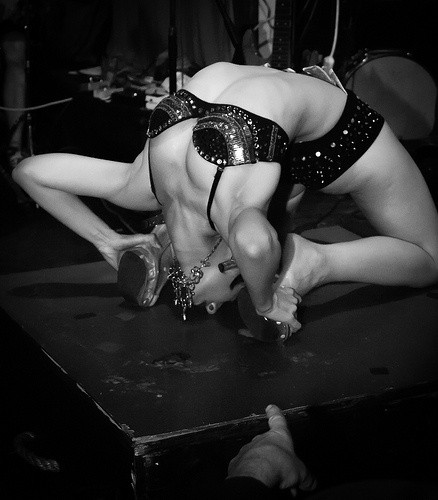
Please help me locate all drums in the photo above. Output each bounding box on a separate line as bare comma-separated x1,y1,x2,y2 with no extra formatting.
342,48,438,150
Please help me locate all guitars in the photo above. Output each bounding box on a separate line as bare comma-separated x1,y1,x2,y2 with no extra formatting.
240,0,299,70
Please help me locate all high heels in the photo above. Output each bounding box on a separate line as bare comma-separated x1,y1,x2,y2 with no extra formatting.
217,256,292,344
119,247,160,309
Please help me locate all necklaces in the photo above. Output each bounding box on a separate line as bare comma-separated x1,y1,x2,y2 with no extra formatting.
168,239,222,318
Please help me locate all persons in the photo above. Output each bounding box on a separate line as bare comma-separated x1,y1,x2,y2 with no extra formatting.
38,43,176,236
12,61,438,349
216,403,321,500
